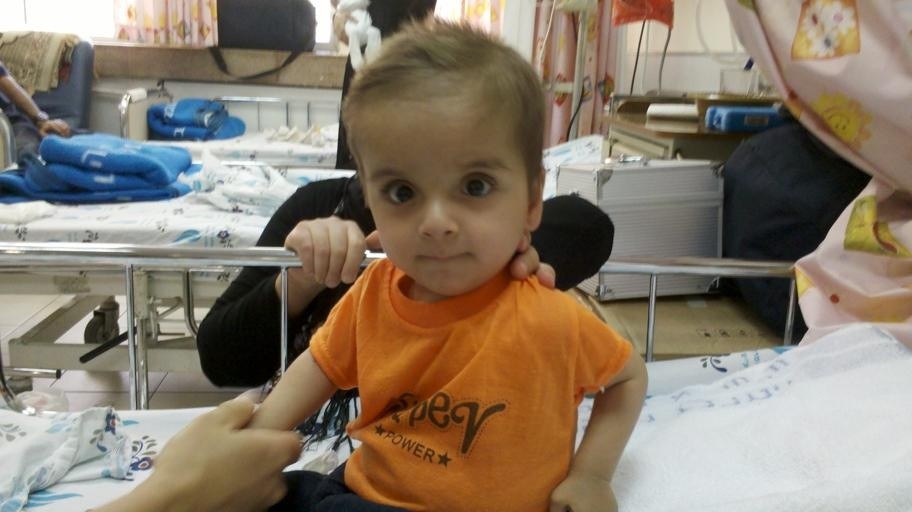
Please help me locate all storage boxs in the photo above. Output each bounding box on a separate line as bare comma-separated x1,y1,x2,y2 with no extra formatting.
556,160,726,304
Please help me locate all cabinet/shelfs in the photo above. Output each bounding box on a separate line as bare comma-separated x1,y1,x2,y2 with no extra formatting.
605,89,781,161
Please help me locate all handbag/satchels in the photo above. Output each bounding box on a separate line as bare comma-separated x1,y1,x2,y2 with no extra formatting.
216,0,316,51
722,123,872,331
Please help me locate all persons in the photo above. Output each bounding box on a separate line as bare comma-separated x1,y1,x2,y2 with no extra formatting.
330,0,381,74
197,170,615,398
0,58,69,140
84,396,301,512
244,12,648,512
333,0,437,169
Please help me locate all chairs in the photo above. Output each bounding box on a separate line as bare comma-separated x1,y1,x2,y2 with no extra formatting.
0,29,94,161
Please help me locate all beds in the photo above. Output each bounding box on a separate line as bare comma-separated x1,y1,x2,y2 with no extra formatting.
119,87,340,165
0,111,637,410
0,242,912,512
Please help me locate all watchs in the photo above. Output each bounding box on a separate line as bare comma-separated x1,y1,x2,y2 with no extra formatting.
33,112,49,125
